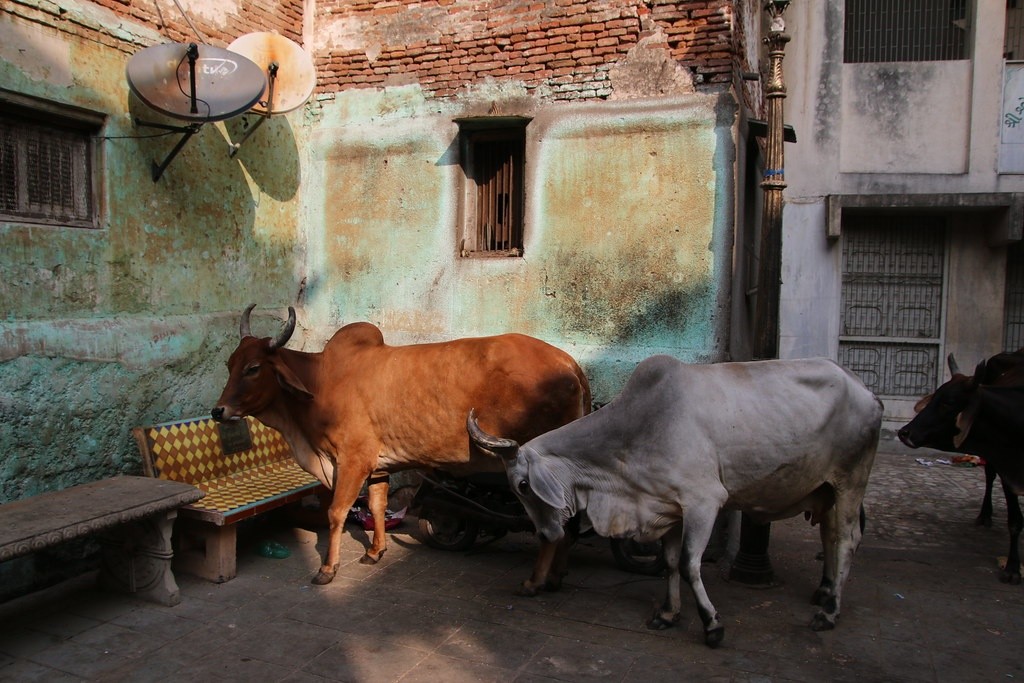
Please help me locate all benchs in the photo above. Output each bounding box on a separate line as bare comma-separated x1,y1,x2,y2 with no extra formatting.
0,474,207,607
132,412,327,582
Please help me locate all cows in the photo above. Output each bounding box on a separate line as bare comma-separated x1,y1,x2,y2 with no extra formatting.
899,346,1024,587
212,303,888,648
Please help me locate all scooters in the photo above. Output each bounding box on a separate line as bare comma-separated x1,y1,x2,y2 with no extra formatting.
416,392,666,574
